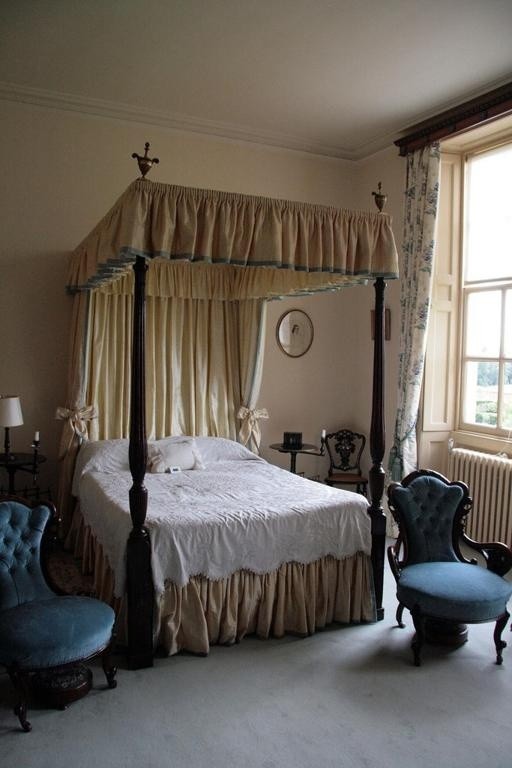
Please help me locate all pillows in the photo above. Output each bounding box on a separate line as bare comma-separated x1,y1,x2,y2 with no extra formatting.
163,436,262,463
148,439,204,472
80,438,129,474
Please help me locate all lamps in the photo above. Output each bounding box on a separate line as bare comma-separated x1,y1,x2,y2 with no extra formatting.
0,395,25,462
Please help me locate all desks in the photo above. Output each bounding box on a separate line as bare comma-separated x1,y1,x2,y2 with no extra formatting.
0,452,47,493
269,443,317,474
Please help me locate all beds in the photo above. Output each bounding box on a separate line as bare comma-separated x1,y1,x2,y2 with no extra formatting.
66,140,399,672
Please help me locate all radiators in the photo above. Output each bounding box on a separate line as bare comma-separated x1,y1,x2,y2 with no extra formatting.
446,436,512,549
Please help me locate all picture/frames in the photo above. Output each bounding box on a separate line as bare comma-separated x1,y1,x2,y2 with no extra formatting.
276,309,314,357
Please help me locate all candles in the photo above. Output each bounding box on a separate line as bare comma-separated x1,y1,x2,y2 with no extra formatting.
34,430,40,442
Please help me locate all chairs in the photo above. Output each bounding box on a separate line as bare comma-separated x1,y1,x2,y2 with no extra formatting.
0,493,117,732
324,430,368,496
387,468,512,668
